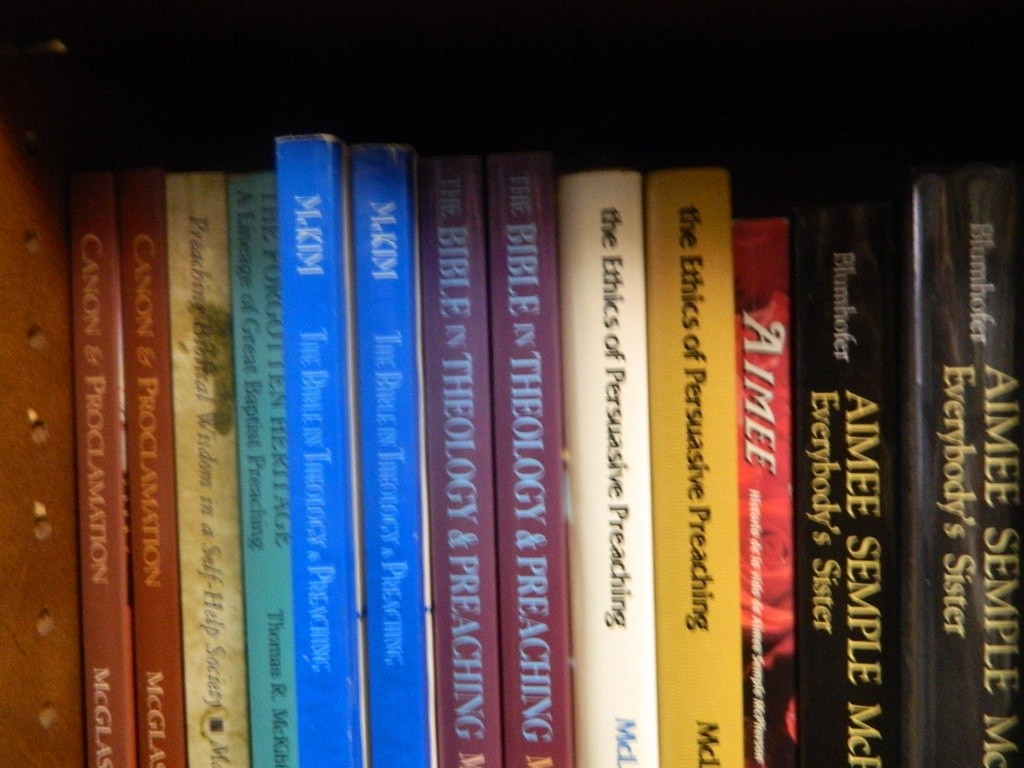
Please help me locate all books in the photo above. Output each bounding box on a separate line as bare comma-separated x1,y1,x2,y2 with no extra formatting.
0,105,1024,768
